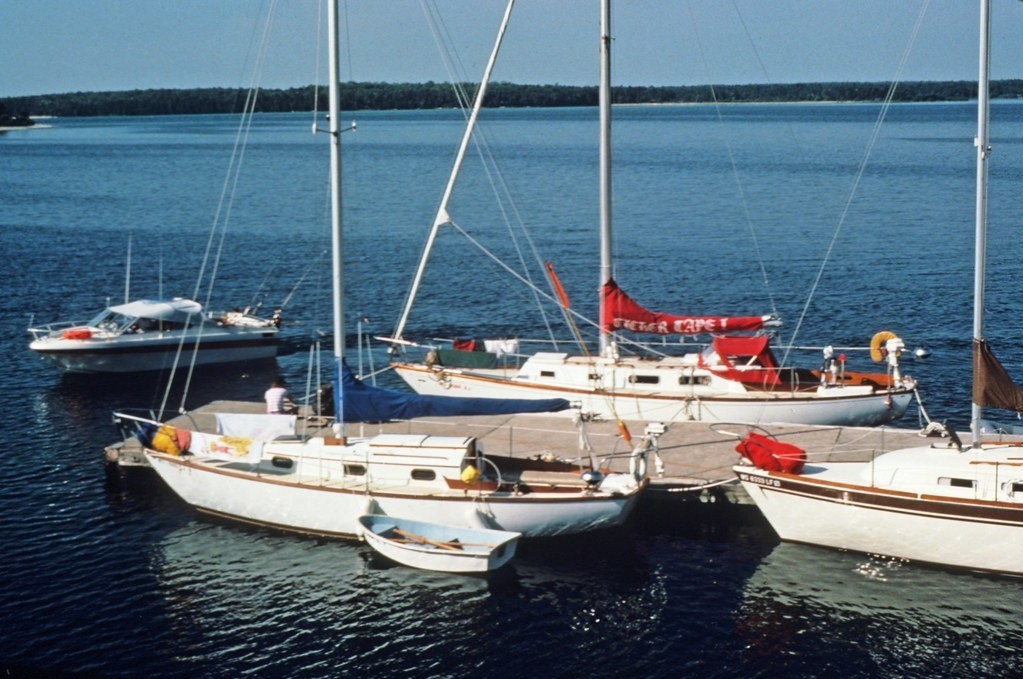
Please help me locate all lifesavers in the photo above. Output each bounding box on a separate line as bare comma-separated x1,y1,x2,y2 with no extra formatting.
870,331,901,362
63,330,92,339
630,447,648,489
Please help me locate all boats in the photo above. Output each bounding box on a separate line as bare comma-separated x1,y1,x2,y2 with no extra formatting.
356,514,522,575
26,230,330,376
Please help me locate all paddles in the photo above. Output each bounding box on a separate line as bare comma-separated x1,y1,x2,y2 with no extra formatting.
388,529,494,551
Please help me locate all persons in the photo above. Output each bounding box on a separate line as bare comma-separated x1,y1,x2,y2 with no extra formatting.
263,378,298,415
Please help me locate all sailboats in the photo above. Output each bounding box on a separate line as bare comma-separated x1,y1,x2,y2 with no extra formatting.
390,2,925,424
103,1,654,543
729,2,1022,585
